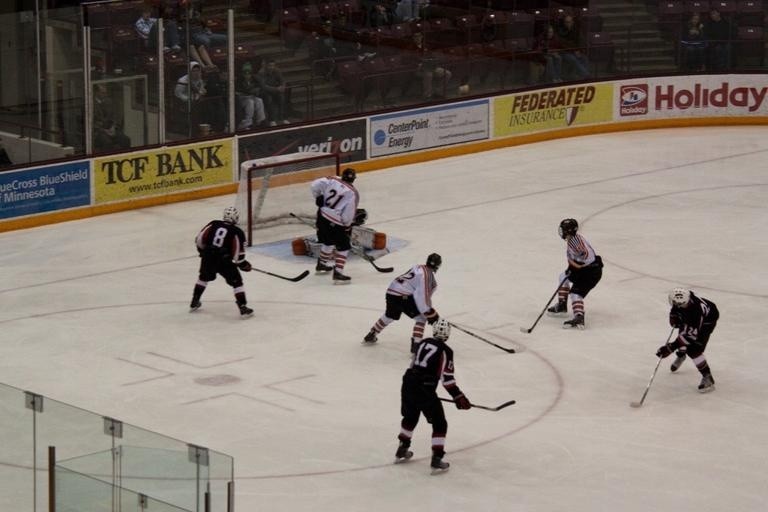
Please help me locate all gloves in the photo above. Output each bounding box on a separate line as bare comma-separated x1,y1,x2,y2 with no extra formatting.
424,309,439,324
317,195,324,206
455,393,471,409
670,316,680,327
239,260,251,272
656,343,675,358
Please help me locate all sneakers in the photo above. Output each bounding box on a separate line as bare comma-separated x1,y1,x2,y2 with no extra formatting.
671,352,686,371
316,258,351,280
698,375,714,388
396,440,413,458
548,303,567,312
240,306,253,314
191,301,201,307
431,449,450,469
564,315,584,325
411,338,419,354
365,331,378,342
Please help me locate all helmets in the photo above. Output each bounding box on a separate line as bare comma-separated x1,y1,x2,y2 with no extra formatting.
558,219,578,239
671,288,690,305
223,207,240,224
432,318,450,341
426,254,442,269
342,168,356,183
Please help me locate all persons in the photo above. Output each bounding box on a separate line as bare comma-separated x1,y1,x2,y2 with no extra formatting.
190,207,253,316
310,168,367,281
548,218,604,327
655,287,719,390
396,319,470,469
678,8,739,74
364,253,442,353
81,0,291,153
360,0,592,101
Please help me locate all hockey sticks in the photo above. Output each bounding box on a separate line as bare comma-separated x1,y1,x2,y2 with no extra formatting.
447,322,515,353
519,276,568,334
631,327,677,409
251,266,309,282
435,399,516,412
352,238,394,273
